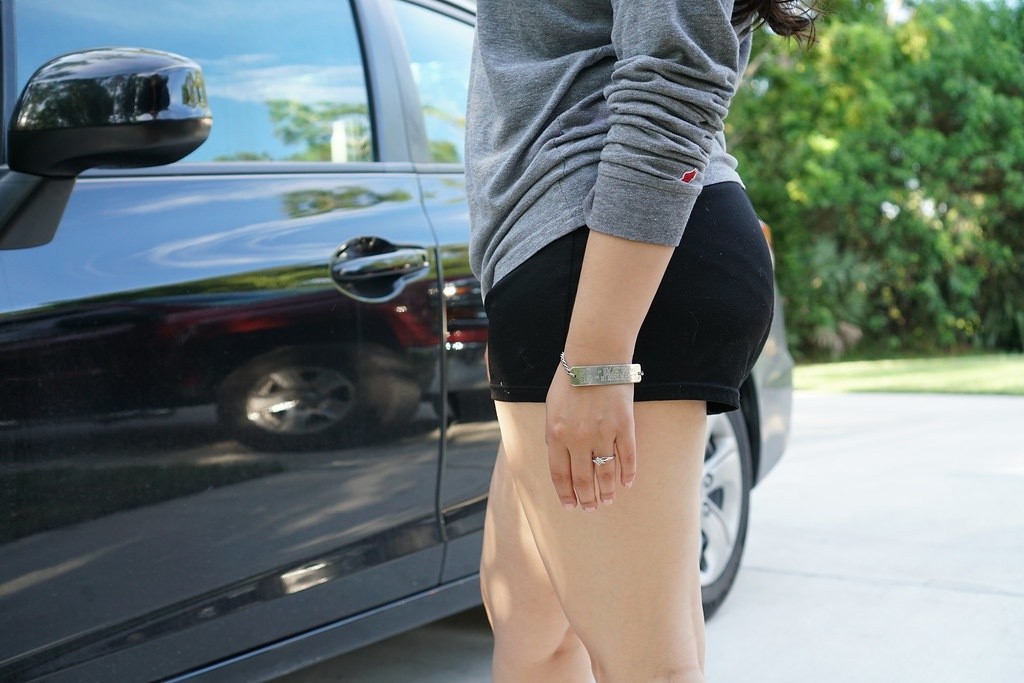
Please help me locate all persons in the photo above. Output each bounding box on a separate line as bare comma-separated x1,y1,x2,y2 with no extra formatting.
464,1,820,683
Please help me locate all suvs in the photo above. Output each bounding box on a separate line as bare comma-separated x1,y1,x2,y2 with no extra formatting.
0,0,795,683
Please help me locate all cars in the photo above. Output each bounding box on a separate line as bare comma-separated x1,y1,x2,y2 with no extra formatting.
43,202,492,452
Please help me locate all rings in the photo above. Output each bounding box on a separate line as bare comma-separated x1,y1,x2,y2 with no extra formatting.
592,453,617,464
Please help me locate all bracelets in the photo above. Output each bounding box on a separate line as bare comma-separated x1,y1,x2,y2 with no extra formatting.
560,352,644,387
